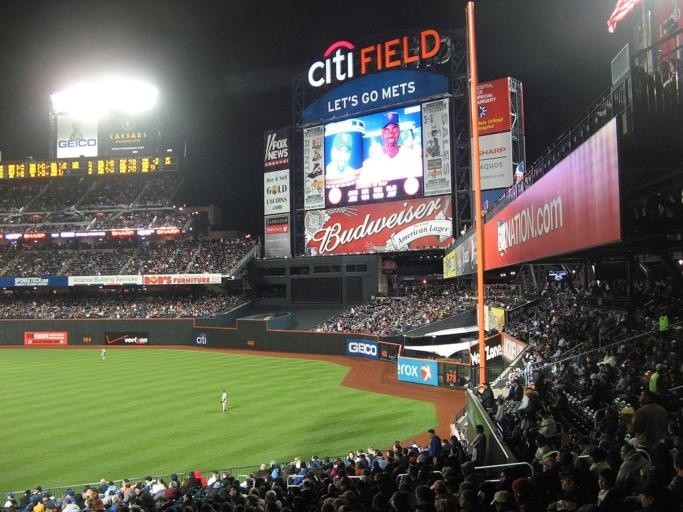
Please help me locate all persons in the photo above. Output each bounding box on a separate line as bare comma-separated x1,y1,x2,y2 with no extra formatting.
220,389,227,412
99,347,106,361
311,278,475,335
0,172,259,319
477,276,683,510
0,427,476,511
324,132,356,180
356,111,423,182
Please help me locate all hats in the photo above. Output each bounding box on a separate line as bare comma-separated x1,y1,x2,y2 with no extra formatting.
489,489,514,506
429,479,445,491
459,461,472,473
557,468,582,481
538,458,554,466
